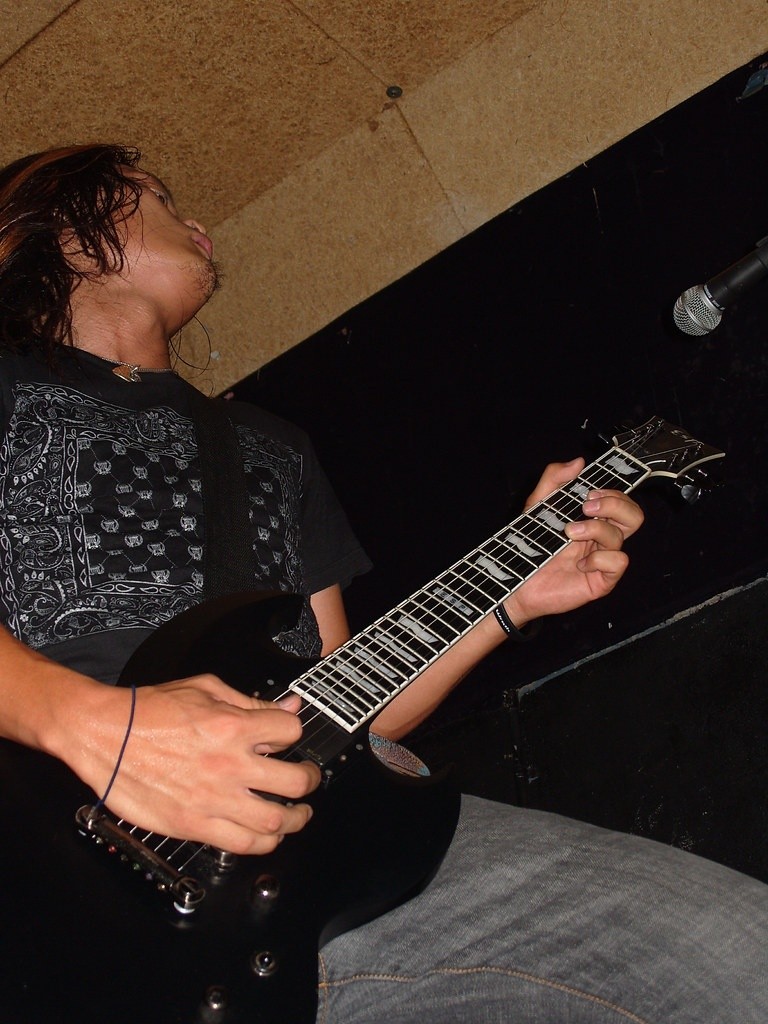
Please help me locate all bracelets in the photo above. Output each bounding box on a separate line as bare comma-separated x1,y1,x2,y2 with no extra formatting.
493,602,543,643
99,685,136,806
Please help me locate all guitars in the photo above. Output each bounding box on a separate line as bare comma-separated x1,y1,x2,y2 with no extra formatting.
0,411,728,1023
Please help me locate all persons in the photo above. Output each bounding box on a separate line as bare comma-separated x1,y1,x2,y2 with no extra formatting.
0,143,644,1024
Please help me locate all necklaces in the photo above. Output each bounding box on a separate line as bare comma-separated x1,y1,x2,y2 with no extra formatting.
65,343,172,383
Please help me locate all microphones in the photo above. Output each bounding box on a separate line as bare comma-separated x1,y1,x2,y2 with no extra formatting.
672,236,768,336
